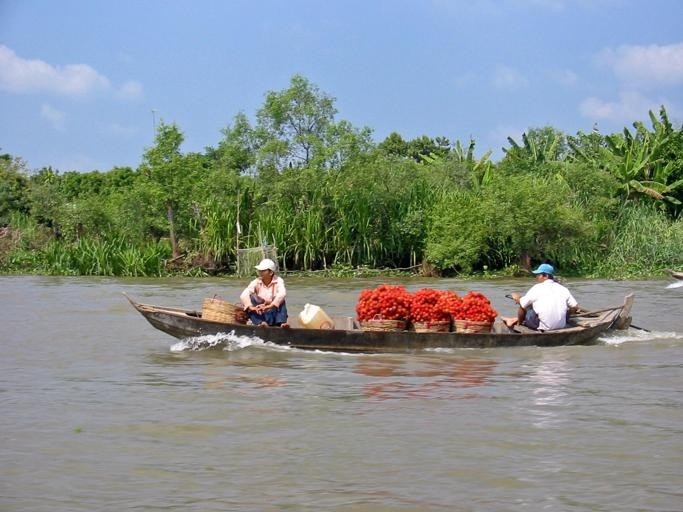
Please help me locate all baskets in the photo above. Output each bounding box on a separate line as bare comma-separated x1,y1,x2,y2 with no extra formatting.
359,318,406,331
411,321,450,333
201,297,249,326
454,320,494,333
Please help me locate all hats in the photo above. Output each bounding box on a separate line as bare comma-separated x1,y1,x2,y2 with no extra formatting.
254,259,276,272
532,264,554,274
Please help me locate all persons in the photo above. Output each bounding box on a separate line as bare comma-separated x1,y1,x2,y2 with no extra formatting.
500,263,581,331
239,258,290,328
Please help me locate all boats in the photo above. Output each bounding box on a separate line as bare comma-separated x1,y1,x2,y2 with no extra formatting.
120,289,635,353
